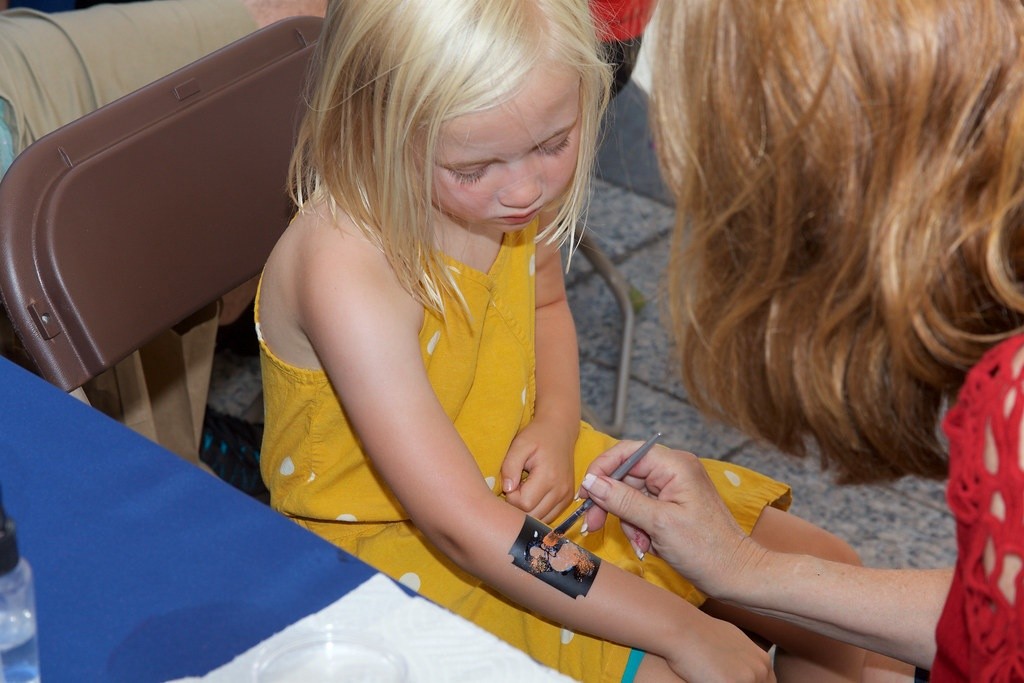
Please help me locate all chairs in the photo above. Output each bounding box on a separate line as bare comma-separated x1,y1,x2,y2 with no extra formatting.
1,15,323,485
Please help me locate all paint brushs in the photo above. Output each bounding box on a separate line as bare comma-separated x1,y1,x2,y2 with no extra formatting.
542,432,664,547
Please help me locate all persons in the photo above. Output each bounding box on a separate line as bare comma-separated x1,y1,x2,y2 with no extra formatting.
253,0,866,682
582,1,654,98
573,0,1023,681
